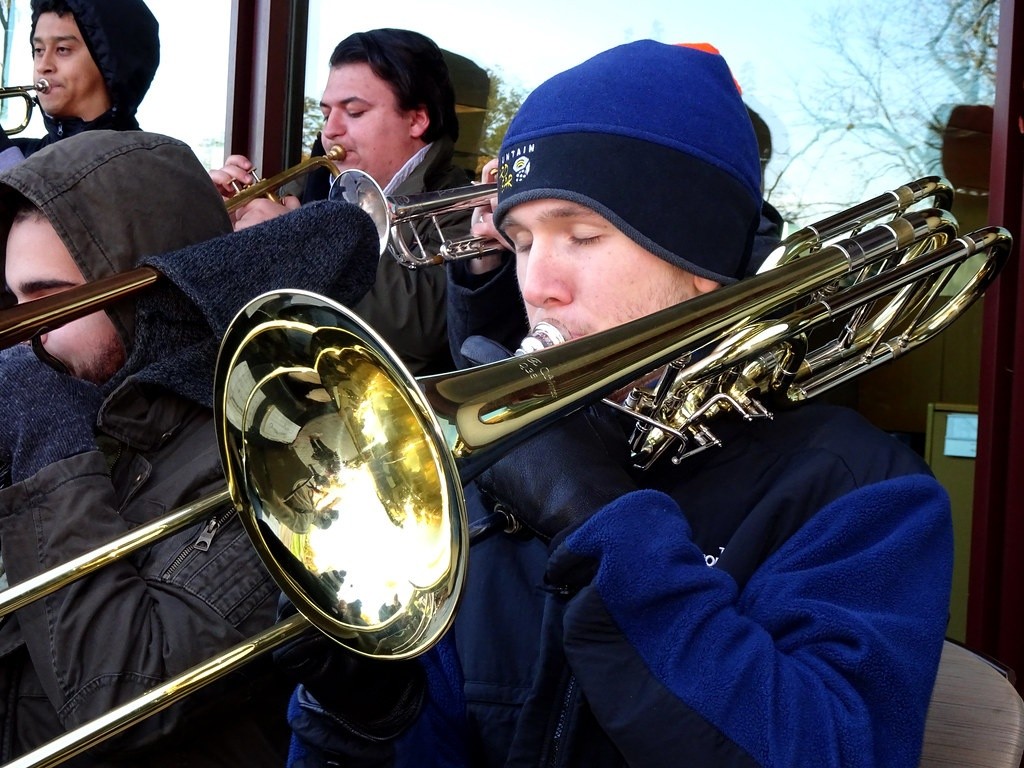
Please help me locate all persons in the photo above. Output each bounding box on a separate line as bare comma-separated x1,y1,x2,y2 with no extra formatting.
445,44,784,371
0,128,282,767
282,39,954,767
0,0,161,175
209,28,479,377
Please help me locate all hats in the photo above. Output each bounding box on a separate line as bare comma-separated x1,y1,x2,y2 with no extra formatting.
492,39,760,284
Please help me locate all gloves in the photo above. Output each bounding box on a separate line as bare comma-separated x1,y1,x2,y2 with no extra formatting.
271,588,429,742
459,334,642,551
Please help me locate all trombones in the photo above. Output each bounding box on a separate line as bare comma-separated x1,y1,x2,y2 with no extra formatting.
0,173,1015,768
0,261,165,358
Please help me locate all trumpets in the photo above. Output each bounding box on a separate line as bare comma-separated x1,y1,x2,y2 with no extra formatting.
323,166,509,274
0,77,54,139
222,141,349,217
